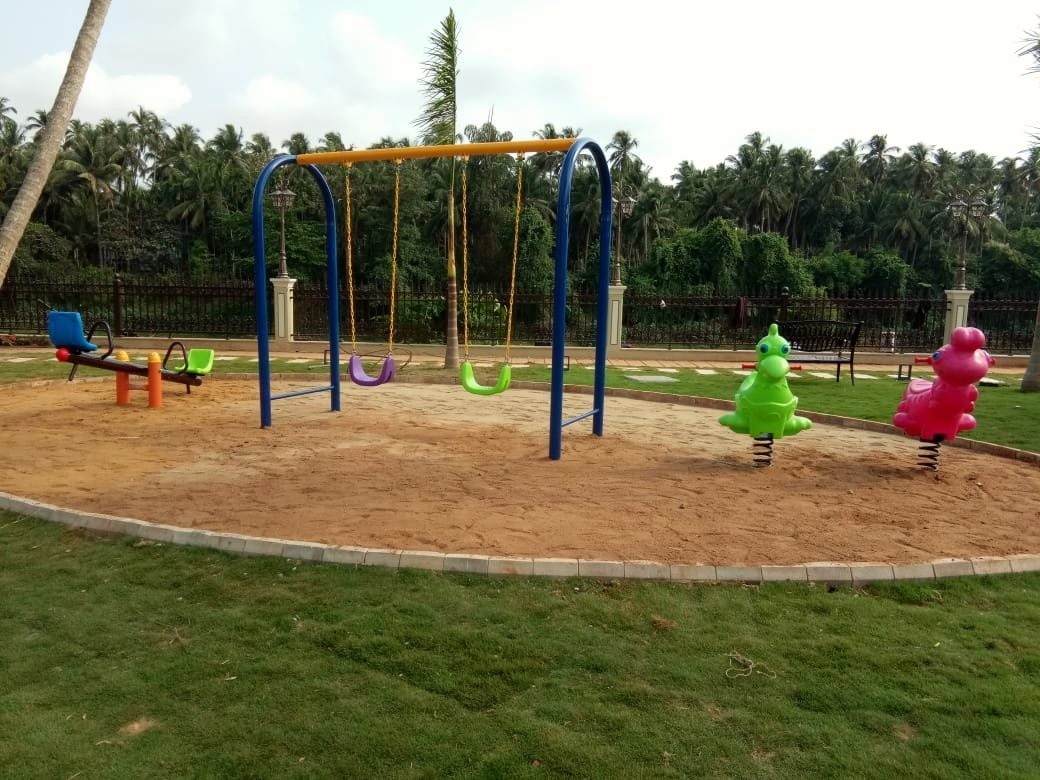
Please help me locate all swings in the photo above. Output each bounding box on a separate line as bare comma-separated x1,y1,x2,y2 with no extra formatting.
456,151,526,396
323,160,404,387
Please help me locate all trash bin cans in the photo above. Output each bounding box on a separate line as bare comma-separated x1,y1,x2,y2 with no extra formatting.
881,331,895,353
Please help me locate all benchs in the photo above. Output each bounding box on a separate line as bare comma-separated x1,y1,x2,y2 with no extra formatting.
776,319,865,386
897,362,931,379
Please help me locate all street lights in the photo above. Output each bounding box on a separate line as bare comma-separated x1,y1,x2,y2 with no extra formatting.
267,178,296,278
610,181,639,286
949,186,990,290
